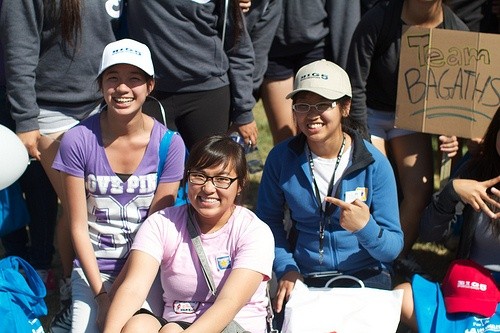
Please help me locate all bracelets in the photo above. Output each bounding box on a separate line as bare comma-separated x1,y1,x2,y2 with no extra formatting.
95,291,107,299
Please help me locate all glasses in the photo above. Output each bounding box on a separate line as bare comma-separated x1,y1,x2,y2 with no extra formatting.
186,169,241,189
292,96,347,113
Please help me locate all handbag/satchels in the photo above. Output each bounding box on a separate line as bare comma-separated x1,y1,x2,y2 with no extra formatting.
0,256,47,333
281,276,405,333
220,320,252,333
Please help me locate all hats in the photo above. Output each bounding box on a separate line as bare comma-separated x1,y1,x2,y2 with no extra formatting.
95,38,155,80
441,259,500,318
285,59,352,100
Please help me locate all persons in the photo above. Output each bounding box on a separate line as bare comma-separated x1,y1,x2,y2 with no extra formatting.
104,136,275,333
51,39,186,333
253,61,402,333
393,108,500,331
0,0,500,313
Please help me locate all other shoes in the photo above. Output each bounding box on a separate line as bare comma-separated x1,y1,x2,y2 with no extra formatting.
394,256,423,280
